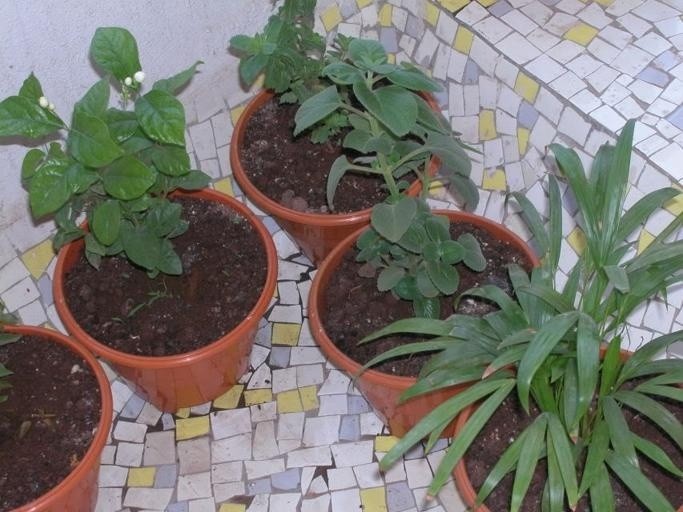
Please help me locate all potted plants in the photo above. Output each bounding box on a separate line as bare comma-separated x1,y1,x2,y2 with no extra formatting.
227,3,448,264
3,26,277,414
292,35,540,445
0,282,115,512
352,121,682,512
